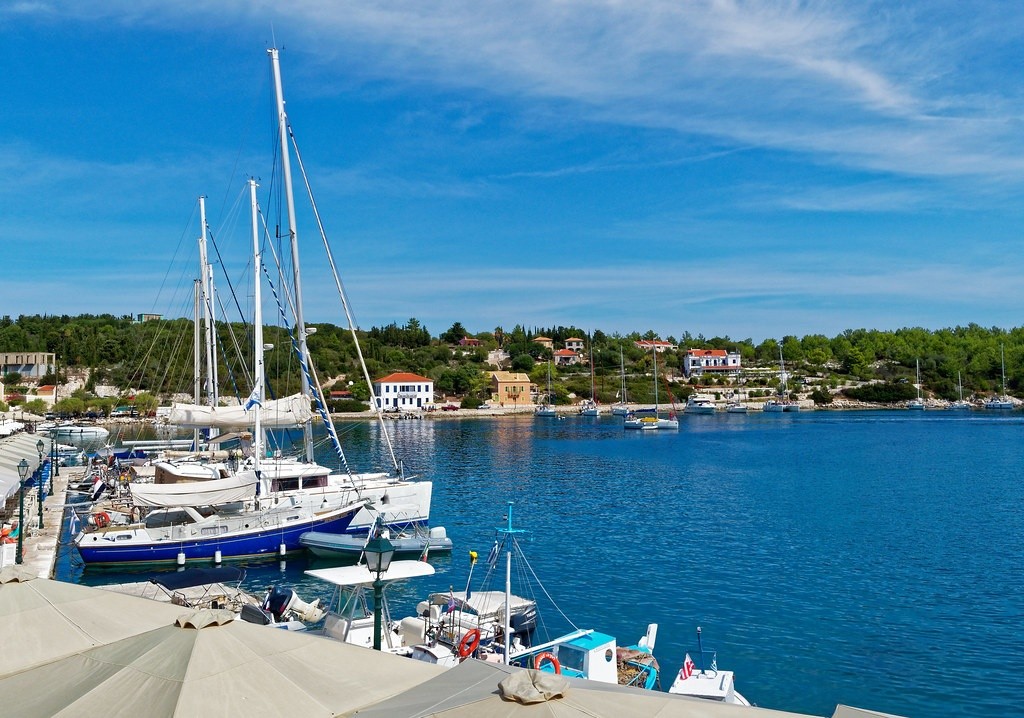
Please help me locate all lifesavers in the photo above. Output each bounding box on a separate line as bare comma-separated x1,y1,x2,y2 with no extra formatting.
534,652,561,674
95,512,109,526
459,628,480,657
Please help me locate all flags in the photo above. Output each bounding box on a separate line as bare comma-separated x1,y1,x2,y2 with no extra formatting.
444,589,457,616
677,652,696,681
89,476,106,501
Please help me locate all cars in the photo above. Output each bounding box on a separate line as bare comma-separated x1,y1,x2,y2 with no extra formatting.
478,404,490,409
45,412,56,421
385,407,397,413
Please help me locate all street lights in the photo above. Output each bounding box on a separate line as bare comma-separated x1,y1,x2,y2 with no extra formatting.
55,425,59,476
36,440,44,528
48,430,55,496
15,459,30,563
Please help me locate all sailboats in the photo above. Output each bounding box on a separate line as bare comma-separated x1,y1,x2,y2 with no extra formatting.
984,350,1014,409
762,347,801,413
34,46,754,711
945,370,976,410
909,357,926,410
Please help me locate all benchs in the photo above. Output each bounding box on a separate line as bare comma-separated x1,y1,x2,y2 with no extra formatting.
401,602,440,640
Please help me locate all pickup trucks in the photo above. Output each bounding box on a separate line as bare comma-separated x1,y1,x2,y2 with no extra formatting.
442,404,458,411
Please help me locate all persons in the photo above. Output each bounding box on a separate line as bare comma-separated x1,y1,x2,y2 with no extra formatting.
5,521,26,564
